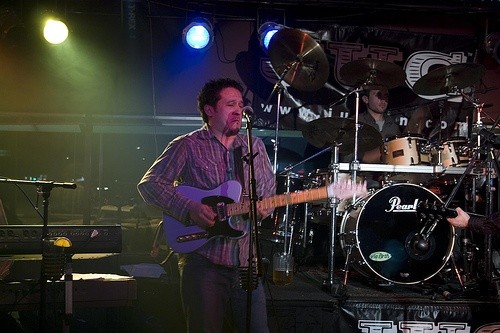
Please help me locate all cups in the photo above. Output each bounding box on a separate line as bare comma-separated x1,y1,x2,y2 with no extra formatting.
273,252,294,286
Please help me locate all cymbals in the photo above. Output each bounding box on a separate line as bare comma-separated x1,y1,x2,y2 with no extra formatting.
267,27,330,92
302,117,383,152
411,61,484,96
338,58,406,90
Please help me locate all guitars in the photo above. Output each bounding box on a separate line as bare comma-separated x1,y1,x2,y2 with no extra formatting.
162,179,369,253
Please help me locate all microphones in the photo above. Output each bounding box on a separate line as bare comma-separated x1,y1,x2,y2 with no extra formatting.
242,106,254,123
412,237,429,251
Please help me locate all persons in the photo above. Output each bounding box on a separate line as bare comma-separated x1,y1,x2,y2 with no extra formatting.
136,78,278,333
446,207,500,237
337,87,403,164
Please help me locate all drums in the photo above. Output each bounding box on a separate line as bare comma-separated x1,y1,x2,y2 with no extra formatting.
435,136,473,167
259,165,320,248
381,132,433,166
336,179,457,291
